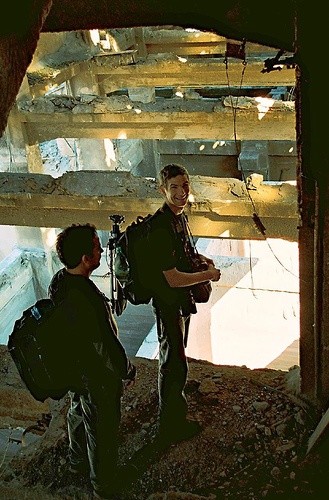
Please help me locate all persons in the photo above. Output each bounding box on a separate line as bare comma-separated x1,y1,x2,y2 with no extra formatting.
124,164,220,432
32,223,139,498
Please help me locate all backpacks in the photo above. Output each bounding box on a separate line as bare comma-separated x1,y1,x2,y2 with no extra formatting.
113,213,152,306
6,298,70,403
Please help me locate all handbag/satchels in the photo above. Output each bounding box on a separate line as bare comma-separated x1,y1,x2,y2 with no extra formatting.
192,255,212,304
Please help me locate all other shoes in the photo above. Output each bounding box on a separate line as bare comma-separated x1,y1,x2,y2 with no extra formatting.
93,469,138,498
155,416,205,441
65,457,92,474
